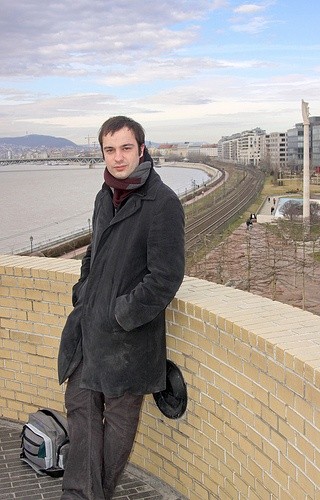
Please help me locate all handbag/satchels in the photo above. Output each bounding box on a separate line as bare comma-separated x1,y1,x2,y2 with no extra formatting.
20,409,72,476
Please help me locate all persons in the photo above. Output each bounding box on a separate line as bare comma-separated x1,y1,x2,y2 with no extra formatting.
245,197,277,232
58,113,188,500
